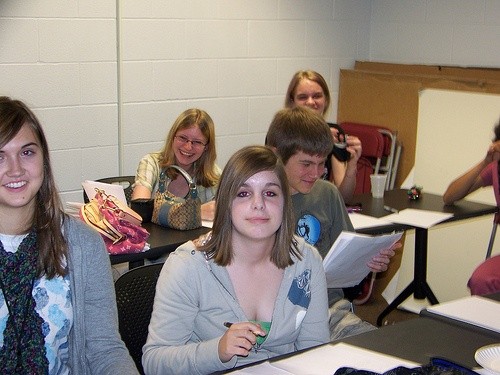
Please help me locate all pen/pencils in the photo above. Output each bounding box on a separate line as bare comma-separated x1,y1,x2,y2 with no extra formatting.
383,204,400,214
347,206,364,212
223,320,261,337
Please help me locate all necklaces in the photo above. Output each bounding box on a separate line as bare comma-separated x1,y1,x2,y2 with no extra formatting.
0,252,45,375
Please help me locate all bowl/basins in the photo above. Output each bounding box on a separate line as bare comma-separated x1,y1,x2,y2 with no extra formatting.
474,343,500,375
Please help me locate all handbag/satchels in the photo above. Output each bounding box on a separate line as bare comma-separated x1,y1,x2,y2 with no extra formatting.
151,165,201,230
79,188,150,255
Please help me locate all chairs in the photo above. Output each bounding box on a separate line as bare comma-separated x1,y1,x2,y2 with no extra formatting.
83,177,135,204
339,122,401,305
114,261,165,375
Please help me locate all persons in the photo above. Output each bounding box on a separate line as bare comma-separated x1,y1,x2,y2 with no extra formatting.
129,109,224,209
443,125,500,298
262,71,362,205
0,96,140,375
141,145,329,375
264,104,404,341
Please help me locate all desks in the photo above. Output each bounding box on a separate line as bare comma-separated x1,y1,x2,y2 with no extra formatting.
208,315,500,375
107,186,499,325
420,293,500,341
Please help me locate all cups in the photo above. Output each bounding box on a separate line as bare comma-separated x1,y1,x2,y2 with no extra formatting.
130,197,154,223
369,173,387,198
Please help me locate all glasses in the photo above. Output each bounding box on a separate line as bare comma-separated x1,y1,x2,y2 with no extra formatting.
174,136,207,148
